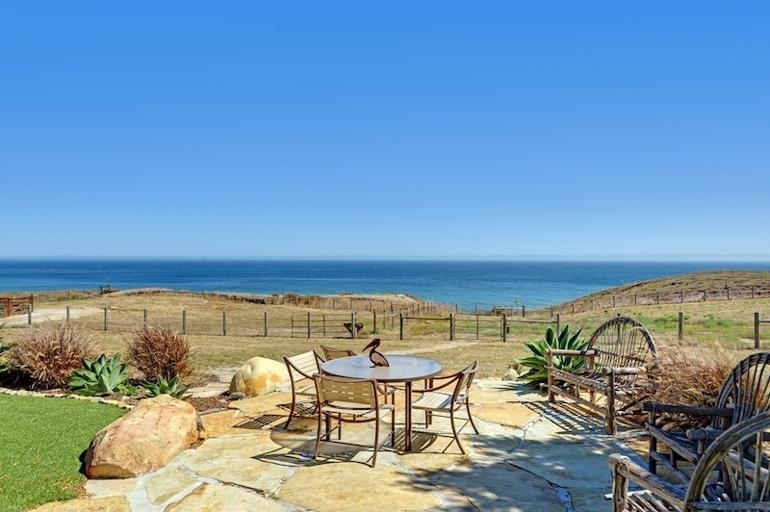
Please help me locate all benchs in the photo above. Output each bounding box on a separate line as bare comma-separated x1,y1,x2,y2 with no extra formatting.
547,315,660,438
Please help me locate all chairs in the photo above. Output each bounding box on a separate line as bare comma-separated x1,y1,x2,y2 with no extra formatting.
282,344,480,468
641,352,769,479
610,406,769,511
